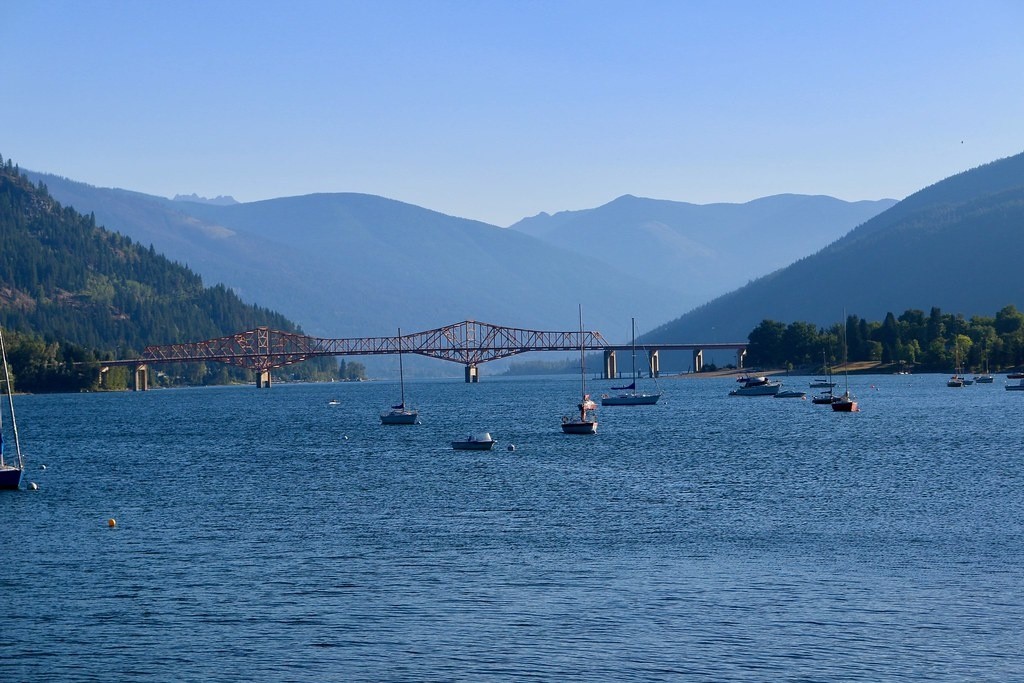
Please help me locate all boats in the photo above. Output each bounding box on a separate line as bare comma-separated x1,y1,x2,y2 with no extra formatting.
329,401,340,405
451,434,496,451
1005,380,1024,390
735,377,750,383
728,374,782,395
1007,372,1024,379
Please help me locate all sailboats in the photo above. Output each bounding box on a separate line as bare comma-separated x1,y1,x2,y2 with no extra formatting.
948,334,974,388
810,349,837,388
832,307,857,412
0,332,23,489
974,339,994,383
812,362,840,404
561,304,598,433
602,318,662,405
380,328,417,424
578,324,599,411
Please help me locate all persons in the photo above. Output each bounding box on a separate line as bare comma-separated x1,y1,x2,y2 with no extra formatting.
579,395,595,422
